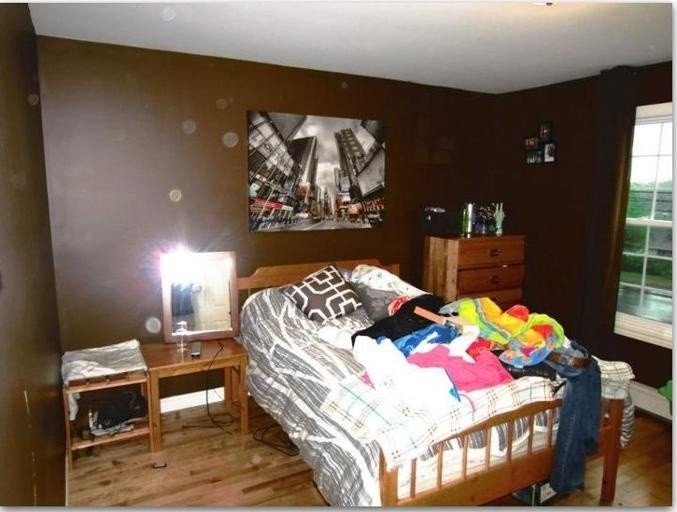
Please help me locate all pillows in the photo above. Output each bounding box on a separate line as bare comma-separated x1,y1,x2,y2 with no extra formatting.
279,262,364,326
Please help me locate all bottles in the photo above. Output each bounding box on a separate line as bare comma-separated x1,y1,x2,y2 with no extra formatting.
524,121,556,164
458,200,506,238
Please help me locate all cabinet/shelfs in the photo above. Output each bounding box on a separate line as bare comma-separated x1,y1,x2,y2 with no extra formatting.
421,235,527,312
62,371,154,471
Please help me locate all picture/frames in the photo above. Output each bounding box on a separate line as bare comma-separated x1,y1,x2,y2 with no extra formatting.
522,120,560,168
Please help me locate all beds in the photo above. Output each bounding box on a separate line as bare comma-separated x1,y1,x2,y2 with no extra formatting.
230,257,634,506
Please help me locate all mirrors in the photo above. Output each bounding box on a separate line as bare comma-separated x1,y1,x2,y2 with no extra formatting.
161,251,240,343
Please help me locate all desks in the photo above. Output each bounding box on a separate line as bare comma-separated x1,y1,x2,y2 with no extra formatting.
141,337,249,452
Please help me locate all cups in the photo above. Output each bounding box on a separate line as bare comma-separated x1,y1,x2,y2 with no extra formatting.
176,348,189,363
175,321,189,348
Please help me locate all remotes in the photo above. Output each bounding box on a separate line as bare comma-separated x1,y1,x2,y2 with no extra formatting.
190,341,202,356
